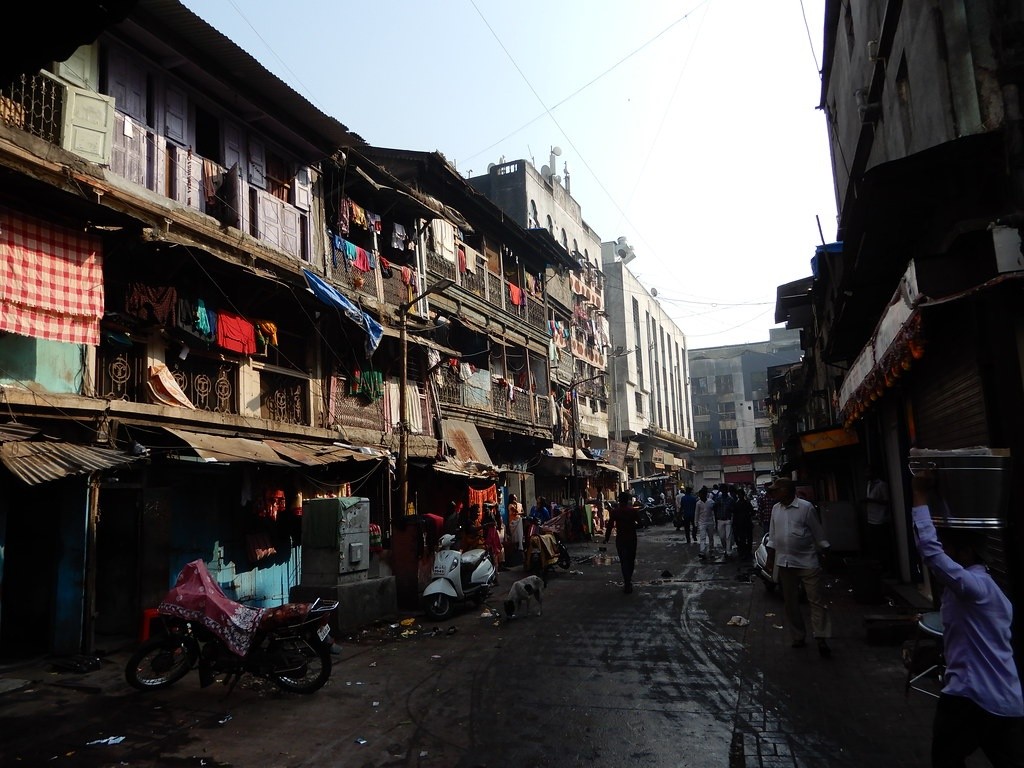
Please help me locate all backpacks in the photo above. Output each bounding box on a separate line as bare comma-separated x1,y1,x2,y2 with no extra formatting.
711,491,720,501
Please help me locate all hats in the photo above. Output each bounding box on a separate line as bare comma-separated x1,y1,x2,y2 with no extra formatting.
617,492,631,499
681,490,684,493
767,477,792,489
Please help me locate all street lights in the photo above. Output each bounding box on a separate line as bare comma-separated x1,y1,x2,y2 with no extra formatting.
388,279,448,611
566,376,602,554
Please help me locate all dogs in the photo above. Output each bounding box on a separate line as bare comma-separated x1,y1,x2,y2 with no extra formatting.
502,574,545,619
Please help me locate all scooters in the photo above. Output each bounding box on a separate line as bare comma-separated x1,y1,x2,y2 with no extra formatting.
425,527,497,621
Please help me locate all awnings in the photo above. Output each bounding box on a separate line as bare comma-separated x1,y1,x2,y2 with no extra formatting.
120,1,367,167
422,150,581,272
1,399,385,486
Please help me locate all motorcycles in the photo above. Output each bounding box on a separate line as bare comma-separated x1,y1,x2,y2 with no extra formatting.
128,559,336,691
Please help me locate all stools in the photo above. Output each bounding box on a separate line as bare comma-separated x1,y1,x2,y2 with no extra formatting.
138,608,161,643
905,614,944,698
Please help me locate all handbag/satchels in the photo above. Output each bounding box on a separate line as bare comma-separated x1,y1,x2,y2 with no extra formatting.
673,515,685,528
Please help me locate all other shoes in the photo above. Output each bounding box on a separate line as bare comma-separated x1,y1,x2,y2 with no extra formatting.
687,540,690,543
792,639,807,649
817,641,832,657
692,536,697,541
709,552,715,558
498,567,511,572
698,553,706,559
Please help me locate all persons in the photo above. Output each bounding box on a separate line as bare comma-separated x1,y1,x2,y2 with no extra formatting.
462,494,558,574
602,492,642,594
857,468,893,525
905,471,1024,768
767,477,834,659
679,479,822,559
